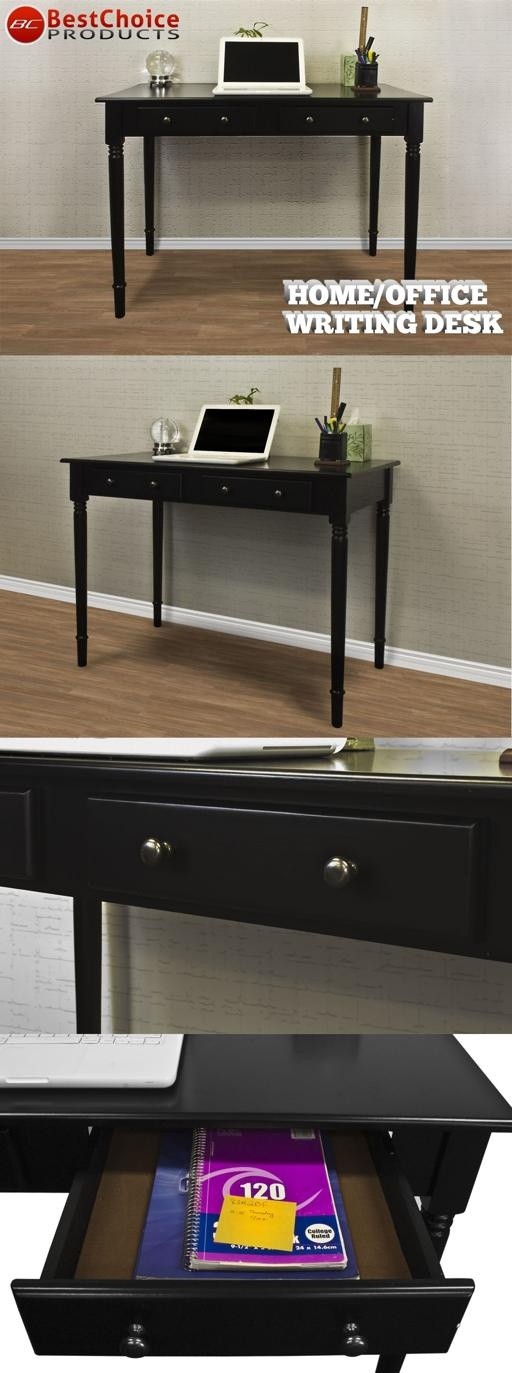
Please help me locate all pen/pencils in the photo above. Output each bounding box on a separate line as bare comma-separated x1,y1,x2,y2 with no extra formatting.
355,37,376,65
315,403,346,434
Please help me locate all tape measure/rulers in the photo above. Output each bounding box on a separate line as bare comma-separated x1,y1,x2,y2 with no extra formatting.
359,8,368,50
331,368,341,416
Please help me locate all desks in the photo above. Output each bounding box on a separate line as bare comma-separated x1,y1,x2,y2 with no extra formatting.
2,738,512,1040
57,442,403,728
97,80,437,319
0,1025,511,1373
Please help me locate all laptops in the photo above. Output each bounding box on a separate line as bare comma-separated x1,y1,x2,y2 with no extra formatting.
210,37,313,97
153,403,281,463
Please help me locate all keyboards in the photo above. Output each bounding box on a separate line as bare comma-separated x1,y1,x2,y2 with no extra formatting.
0,1032,184,1089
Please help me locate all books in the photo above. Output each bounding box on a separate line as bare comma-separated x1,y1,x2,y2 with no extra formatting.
132,1130,360,1283
182,1128,348,1272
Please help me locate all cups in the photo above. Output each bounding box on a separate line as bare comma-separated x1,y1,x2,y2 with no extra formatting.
319,431,348,460
356,62,380,86
